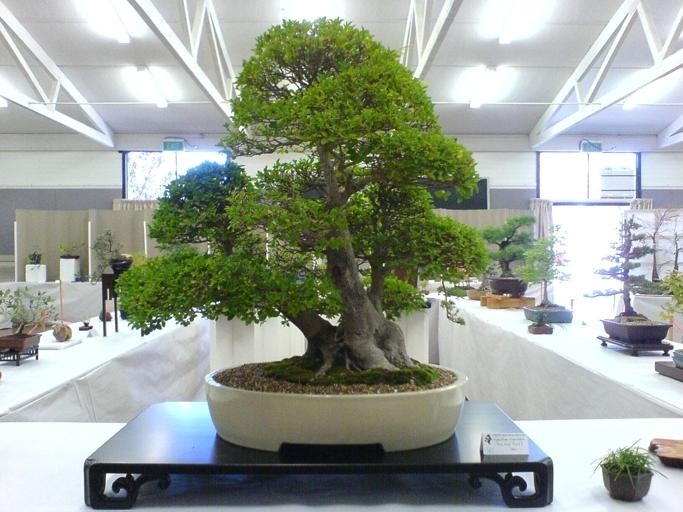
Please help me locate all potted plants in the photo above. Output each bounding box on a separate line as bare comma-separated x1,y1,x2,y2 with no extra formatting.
479,208,681,386
592,444,668,504
0,231,133,368
116,15,490,449
